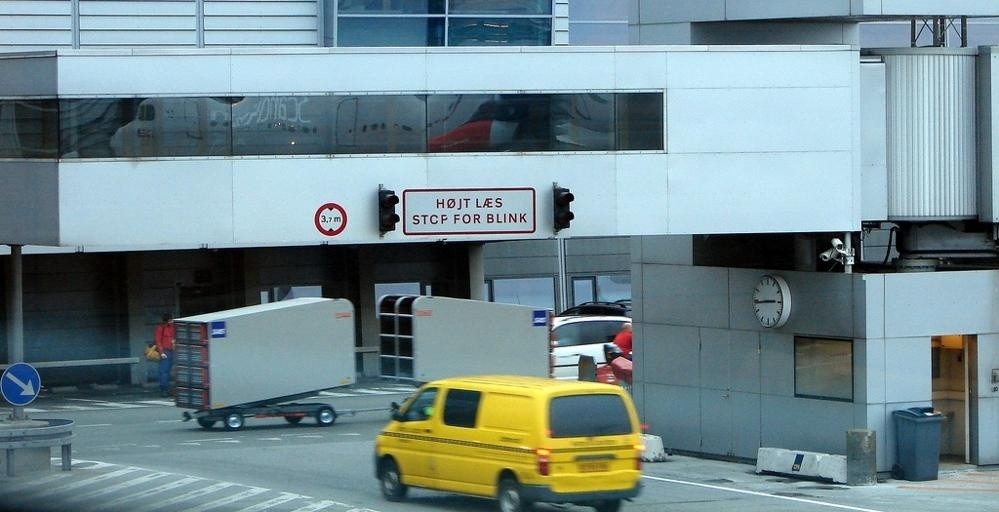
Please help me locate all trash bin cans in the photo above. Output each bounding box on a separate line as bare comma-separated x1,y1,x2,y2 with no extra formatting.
891,407,948,480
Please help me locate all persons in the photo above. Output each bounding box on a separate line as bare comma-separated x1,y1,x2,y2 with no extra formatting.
612,320,633,359
155,312,175,399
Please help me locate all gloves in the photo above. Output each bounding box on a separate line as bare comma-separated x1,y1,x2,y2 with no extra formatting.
160,353,168,358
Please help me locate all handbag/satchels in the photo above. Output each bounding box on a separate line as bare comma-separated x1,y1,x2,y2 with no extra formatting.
145,343,161,362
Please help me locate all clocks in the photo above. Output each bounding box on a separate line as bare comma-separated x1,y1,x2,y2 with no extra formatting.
752,274,792,329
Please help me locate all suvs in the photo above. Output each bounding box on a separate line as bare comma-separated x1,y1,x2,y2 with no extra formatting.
548,296,633,316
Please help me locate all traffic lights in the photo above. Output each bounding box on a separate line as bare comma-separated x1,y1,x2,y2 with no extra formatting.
554,185,573,234
380,188,400,233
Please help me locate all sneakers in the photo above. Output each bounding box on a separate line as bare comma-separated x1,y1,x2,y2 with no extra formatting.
161,388,172,397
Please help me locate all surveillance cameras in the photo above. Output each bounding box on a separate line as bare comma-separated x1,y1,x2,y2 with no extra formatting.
819,248,840,262
831,238,843,251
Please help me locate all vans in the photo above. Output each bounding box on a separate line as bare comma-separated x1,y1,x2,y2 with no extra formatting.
376,377,647,511
